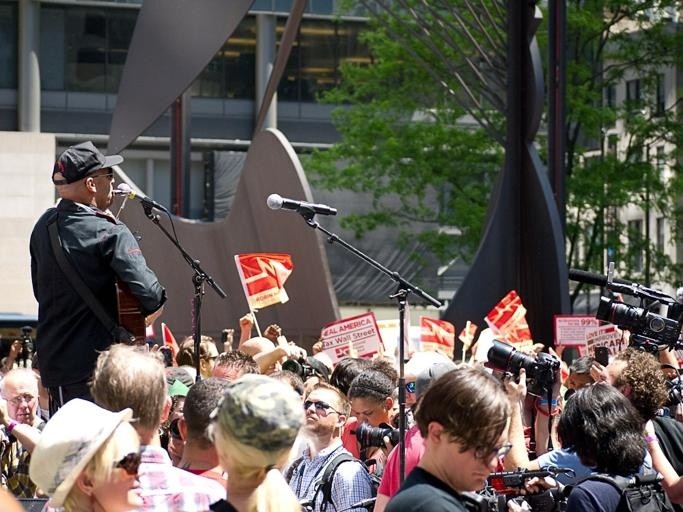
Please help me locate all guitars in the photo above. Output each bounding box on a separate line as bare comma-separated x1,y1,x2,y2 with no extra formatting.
113,282,145,347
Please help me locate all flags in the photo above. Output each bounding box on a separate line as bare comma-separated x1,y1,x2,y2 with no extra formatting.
422,316,455,358
234,252,293,310
486,289,534,350
459,322,478,346
162,322,180,352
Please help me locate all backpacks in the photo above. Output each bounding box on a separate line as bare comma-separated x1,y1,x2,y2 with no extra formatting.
576,461,675,512
321,453,381,512
653,414,683,476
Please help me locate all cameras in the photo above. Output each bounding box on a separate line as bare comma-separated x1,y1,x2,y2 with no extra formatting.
17,326,34,363
661,378,682,407
281,357,314,383
483,339,559,398
355,423,408,451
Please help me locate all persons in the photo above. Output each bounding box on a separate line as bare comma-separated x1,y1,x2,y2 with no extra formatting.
29,139,166,418
2,311,683,511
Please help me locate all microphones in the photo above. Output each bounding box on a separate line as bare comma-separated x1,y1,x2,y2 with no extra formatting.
266,194,337,216
116,181,167,213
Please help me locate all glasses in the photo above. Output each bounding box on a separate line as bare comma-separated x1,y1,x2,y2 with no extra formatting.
304,401,344,414
117,453,139,474
93,168,113,178
460,435,512,459
406,381,416,392
3,395,41,402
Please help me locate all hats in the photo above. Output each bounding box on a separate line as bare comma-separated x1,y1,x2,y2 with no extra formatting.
29,398,133,508
296,356,328,381
52,141,124,184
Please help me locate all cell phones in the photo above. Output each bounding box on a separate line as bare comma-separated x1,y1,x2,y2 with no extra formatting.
594,346,608,367
220,330,227,342
